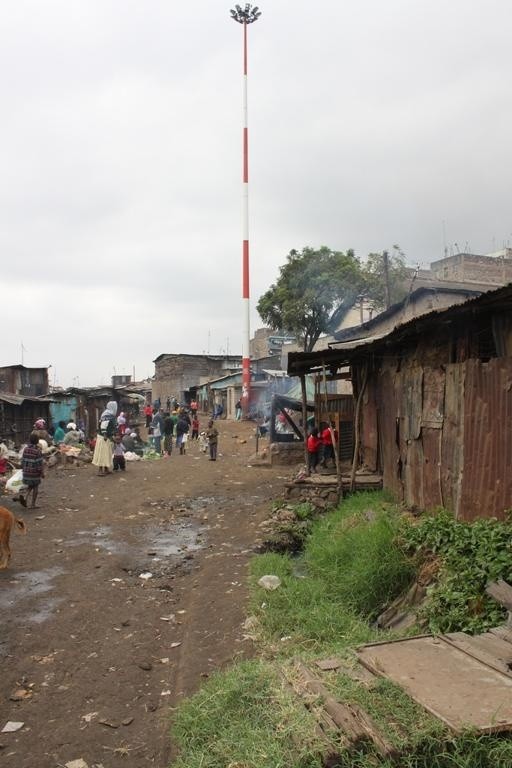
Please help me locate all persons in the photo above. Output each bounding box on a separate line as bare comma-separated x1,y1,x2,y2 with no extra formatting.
212,404,223,420
0,437,10,474
308,429,320,473
17,429,46,509
320,420,337,468
235,398,242,421
22,391,218,478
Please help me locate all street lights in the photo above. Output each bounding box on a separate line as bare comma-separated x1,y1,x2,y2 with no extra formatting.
227,0,263,423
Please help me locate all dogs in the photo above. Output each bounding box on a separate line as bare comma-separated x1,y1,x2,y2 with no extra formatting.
0,505,27,569
